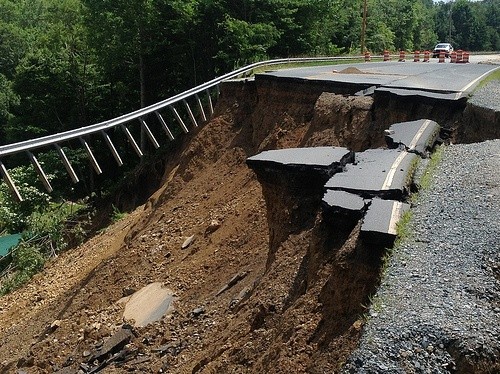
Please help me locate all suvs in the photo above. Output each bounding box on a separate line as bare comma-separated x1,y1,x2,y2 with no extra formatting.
433,43,453,58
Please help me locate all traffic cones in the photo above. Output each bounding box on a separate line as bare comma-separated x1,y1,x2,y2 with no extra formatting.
365,50,469,63
